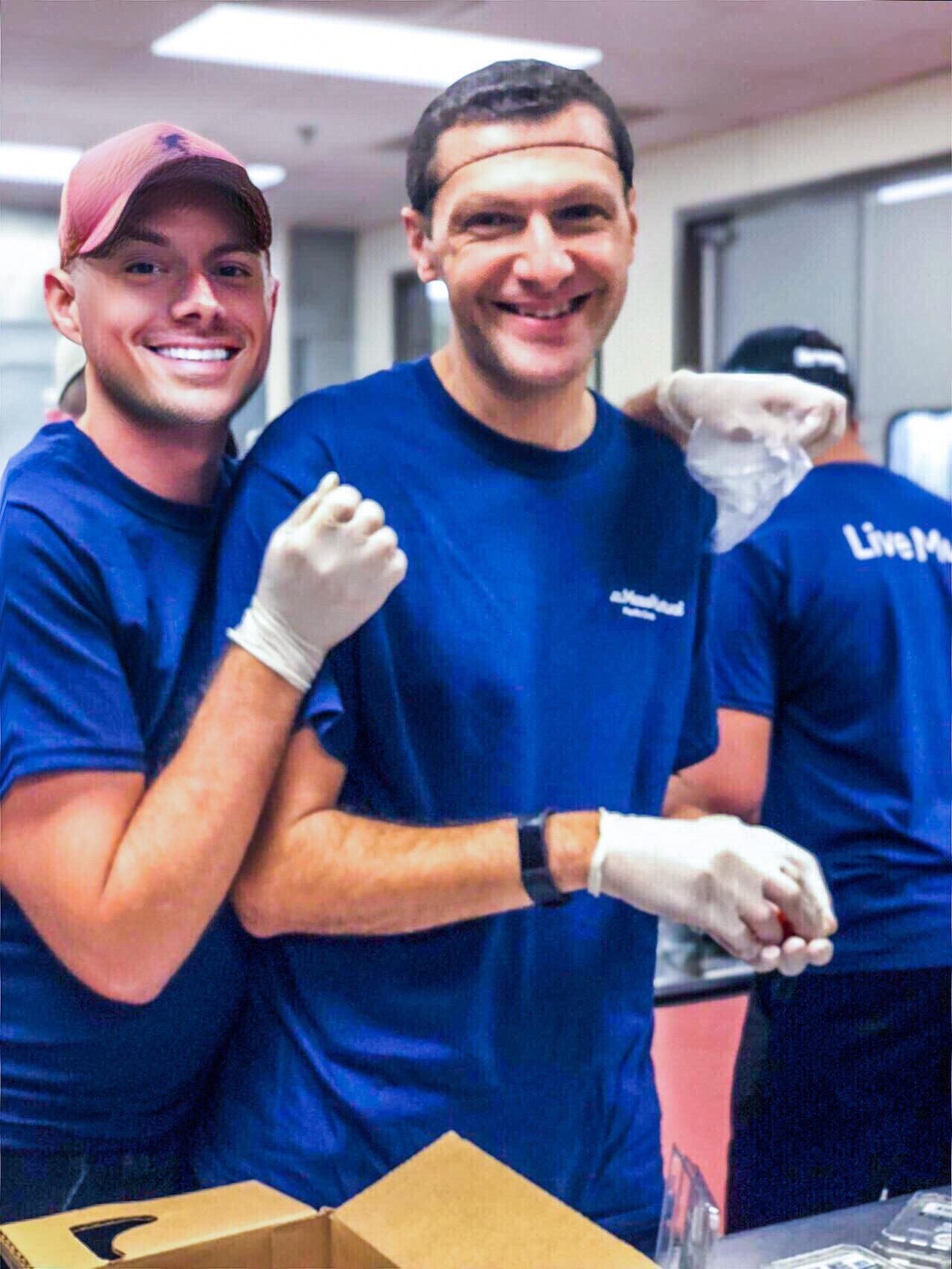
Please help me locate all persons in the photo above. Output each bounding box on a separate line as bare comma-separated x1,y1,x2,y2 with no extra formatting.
208,58,838,1261
0,120,848,1269
662,326,952,1235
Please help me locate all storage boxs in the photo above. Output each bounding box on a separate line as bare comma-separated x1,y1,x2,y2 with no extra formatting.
0,1119,659,1269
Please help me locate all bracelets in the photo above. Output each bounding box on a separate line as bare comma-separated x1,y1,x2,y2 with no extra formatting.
517,807,571,909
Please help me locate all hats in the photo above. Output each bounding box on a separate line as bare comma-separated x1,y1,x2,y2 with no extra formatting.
58,123,272,270
723,326,858,403
